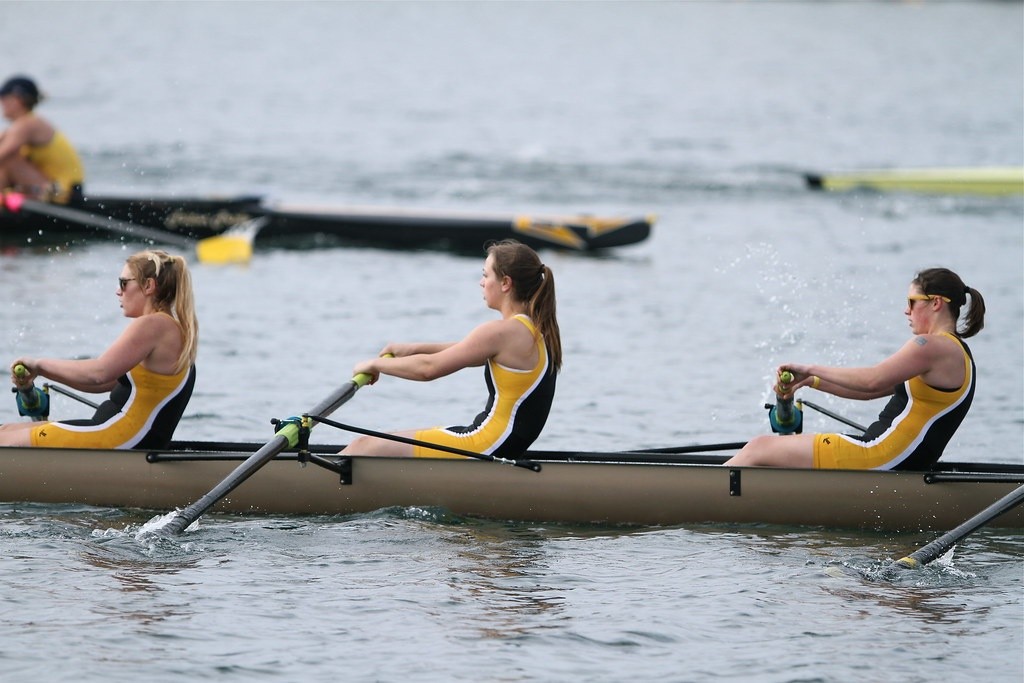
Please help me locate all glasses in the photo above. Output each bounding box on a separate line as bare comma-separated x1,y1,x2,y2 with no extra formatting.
907,294,951,310
118,277,135,291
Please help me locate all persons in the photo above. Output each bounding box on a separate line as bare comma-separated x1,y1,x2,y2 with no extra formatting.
722,268,986,473
0,78,85,217
0,249,199,448
336,238,563,461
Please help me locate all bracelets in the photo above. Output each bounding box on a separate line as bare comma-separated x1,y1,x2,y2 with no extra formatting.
809,376,819,388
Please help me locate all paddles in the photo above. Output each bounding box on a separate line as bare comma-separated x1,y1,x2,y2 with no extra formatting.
160,349,396,534
768,370,801,436
0,193,251,266
11,360,53,420
883,485,1024,575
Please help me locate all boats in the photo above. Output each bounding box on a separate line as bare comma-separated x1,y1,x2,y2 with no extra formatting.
800,164,1024,198
0,185,660,258
0,417,1024,545
